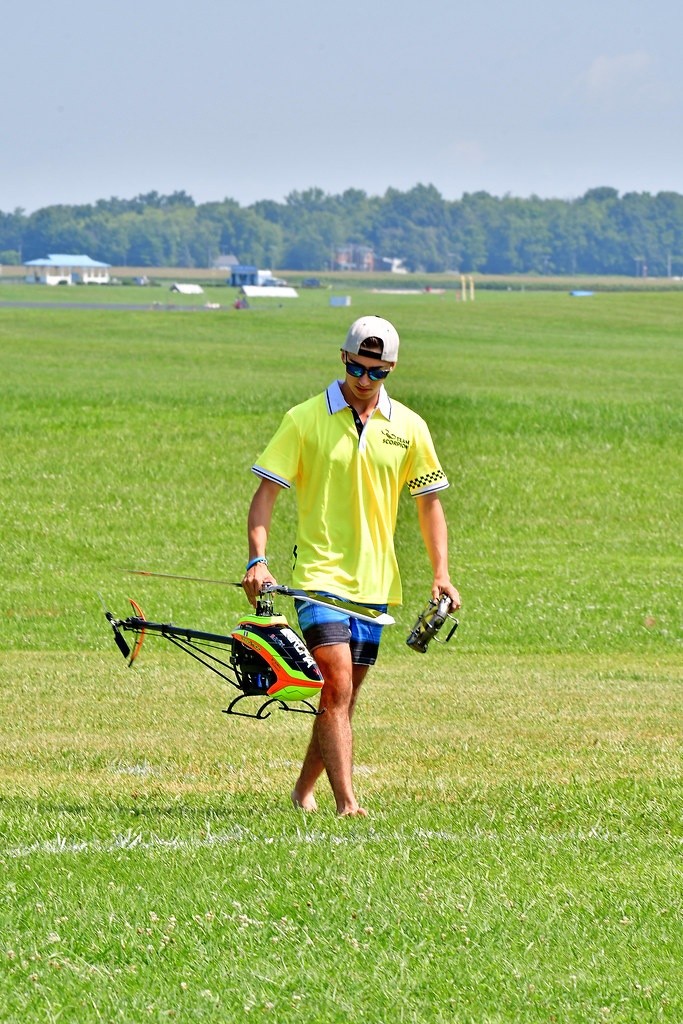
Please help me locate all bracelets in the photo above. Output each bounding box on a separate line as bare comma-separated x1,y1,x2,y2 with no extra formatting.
246,557,267,571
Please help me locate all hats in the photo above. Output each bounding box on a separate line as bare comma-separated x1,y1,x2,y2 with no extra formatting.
341,315,400,363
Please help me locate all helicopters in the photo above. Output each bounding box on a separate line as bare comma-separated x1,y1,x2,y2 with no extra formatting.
105,569,395,720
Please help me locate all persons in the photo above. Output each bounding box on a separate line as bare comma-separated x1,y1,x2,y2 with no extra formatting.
241,315,461,819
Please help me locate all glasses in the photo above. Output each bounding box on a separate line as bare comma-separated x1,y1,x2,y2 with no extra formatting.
344,351,392,382
